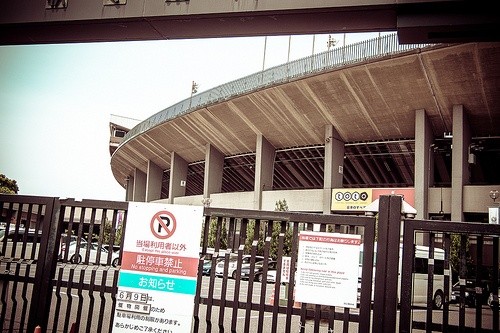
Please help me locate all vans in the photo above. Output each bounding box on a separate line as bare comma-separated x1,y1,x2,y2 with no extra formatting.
356,242,452,311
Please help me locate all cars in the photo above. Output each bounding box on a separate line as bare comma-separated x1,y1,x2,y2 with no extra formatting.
198,247,285,284
0,222,120,268
449,280,498,308
